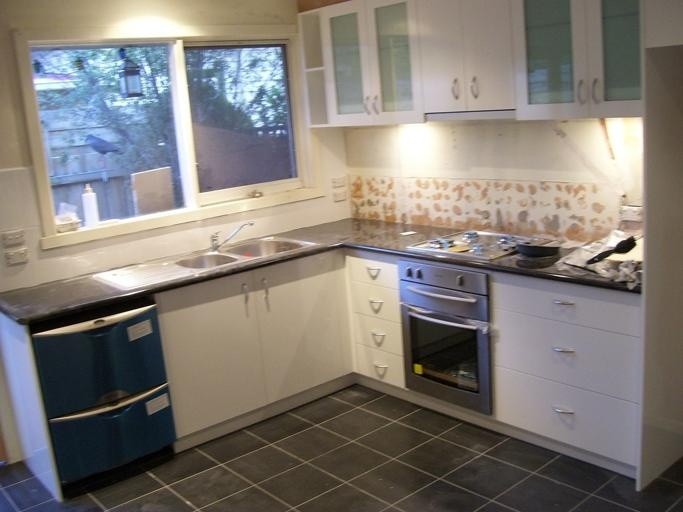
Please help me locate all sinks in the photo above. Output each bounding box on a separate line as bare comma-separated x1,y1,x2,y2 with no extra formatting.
227,235,321,258
174,250,237,271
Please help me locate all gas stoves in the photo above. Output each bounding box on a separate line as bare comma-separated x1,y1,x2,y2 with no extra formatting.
399,230,533,297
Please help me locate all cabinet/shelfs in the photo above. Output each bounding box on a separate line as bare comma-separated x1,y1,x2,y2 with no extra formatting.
512,0,647,122
489,267,642,485
345,240,401,403
297,1,422,127
150,241,350,457
421,0,515,123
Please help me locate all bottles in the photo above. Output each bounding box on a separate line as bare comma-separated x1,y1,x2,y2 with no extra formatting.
81,183,99,228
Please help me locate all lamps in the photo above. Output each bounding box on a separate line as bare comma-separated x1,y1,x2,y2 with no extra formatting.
118,55,145,101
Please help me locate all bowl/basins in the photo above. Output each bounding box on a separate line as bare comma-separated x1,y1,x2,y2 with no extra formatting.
518,242,561,258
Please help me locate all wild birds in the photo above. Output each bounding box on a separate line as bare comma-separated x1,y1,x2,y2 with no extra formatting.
85,134,125,160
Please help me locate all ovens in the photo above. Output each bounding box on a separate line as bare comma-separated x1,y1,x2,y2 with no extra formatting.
399,281,491,417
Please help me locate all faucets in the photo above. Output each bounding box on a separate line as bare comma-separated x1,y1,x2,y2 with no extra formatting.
210,221,254,251
